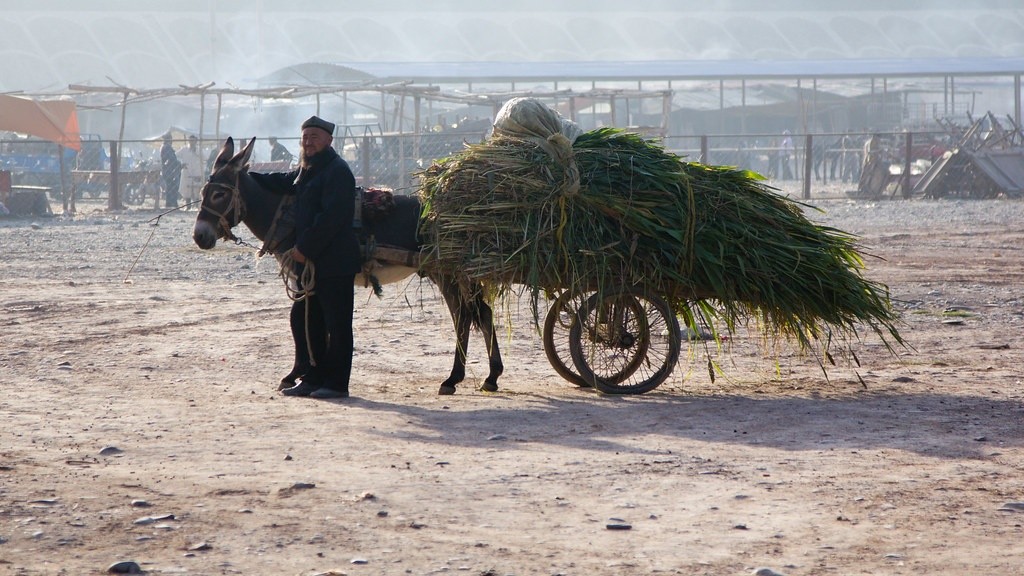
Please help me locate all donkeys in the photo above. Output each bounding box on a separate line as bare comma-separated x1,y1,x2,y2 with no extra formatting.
812,134,847,182
193,136,504,398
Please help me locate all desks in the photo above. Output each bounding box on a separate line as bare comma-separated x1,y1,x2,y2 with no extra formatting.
10,185,54,215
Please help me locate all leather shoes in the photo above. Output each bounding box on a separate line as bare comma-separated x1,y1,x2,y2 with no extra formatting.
310,387,349,399
281,381,319,396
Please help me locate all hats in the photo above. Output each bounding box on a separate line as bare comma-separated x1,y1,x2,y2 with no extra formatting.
302,116,335,135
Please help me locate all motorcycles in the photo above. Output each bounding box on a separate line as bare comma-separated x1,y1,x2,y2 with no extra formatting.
122,152,163,205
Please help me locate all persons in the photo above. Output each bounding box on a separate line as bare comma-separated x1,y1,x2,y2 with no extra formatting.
175,135,204,210
161,132,181,208
267,136,292,162
281,116,357,398
768,139,779,178
780,129,794,180
841,126,904,183
359,137,381,160
